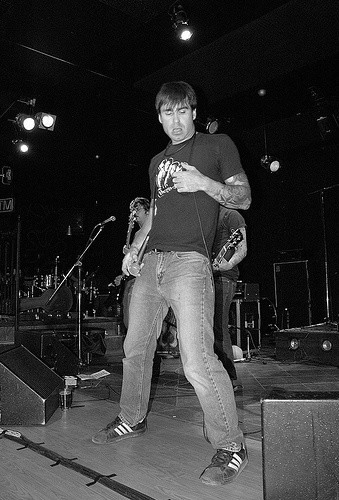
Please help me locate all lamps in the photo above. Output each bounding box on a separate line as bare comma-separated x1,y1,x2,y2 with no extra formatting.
14,112,56,133
167,1,192,41
259,154,280,174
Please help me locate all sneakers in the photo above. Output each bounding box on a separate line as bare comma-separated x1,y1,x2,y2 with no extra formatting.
91,417,146,444
201,443,249,487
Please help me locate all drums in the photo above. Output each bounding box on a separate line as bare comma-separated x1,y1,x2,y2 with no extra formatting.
39,276,72,314
18,286,43,319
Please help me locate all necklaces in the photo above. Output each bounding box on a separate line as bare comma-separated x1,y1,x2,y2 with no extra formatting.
163,131,196,157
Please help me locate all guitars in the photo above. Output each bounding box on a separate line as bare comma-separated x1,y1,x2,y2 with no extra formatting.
213,229,243,264
115,210,138,316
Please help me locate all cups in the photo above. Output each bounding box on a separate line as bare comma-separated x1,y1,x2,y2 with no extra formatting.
59,388,72,412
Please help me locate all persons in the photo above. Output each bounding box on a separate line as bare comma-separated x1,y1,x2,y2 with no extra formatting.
210,204,248,393
114,195,150,329
91,80,252,485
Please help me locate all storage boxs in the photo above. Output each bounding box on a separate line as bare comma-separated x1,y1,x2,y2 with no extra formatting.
229,298,261,356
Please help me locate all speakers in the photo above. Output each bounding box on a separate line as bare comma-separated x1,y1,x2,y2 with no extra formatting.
259,391,339,500
15,330,90,377
229,299,261,355
0,344,65,426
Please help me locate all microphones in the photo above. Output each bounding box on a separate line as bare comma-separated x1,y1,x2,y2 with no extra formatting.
98,216,116,225
